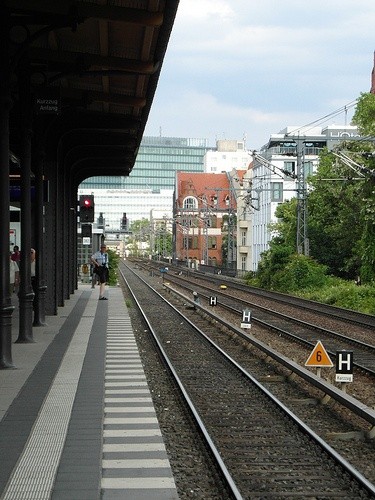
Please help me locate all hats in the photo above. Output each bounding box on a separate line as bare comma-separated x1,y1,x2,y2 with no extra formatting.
100,244,106,248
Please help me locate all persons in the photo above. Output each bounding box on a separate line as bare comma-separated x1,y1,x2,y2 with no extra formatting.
9,251,19,298
30,248,36,311
10,245,21,293
91,244,110,300
90,261,97,288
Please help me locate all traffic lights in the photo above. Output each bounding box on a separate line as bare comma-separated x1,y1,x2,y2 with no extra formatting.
79,195,94,223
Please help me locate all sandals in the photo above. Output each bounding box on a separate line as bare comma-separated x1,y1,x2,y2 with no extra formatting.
99,297,107,300
